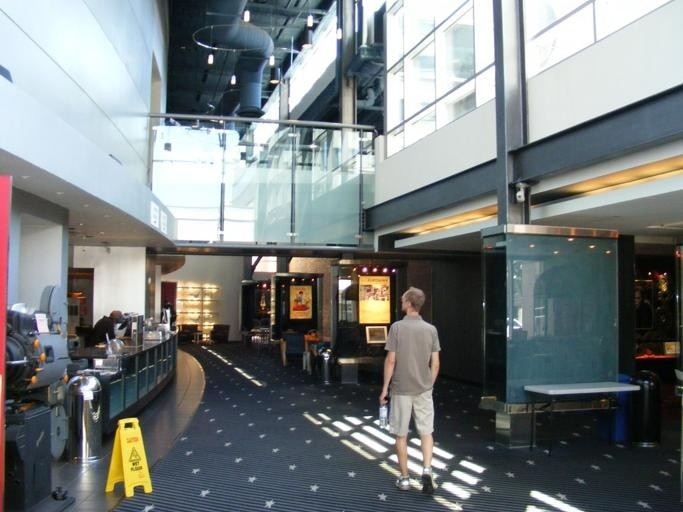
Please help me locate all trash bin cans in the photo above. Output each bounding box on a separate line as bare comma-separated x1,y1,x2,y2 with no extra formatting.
316,347,337,386
631,370,660,448
66,375,103,463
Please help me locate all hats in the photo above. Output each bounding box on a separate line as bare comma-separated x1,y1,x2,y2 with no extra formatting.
109,310,123,319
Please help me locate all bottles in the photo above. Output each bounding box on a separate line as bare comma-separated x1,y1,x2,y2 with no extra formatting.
380,404,387,427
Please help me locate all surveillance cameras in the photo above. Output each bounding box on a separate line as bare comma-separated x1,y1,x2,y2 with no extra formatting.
516,190,525,203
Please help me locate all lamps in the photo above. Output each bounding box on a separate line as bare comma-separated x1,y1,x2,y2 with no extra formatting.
206,3,317,85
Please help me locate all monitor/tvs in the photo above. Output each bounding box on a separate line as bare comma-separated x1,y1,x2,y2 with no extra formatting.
365,326,388,344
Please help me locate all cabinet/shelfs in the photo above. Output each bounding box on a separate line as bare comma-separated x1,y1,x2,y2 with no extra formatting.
481,227,633,450
244,257,410,381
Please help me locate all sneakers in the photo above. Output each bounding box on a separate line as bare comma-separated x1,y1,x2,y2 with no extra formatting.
421,467,435,493
395,474,411,491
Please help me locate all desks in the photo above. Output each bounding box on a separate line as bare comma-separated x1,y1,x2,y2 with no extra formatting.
523,379,642,460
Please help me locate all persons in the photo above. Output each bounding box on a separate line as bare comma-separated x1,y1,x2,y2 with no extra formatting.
293,288,307,305
632,287,653,335
377,285,440,491
91,310,126,345
160,297,177,325
